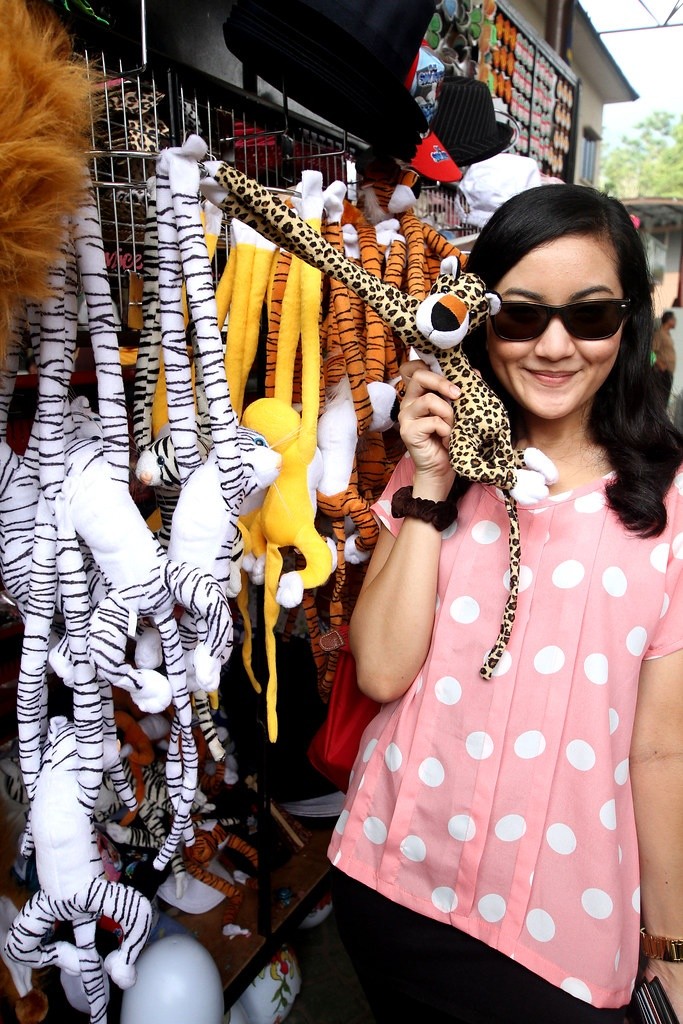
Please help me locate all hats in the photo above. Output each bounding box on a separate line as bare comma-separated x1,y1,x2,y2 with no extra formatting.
432,77,514,167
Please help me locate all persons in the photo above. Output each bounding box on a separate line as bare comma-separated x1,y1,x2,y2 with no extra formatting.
327,185,683,1024
652,311,676,408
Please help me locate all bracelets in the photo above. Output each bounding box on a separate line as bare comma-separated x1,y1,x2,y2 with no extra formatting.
391,485,458,531
640,928,683,963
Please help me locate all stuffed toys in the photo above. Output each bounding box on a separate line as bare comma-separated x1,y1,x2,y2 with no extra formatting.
200,161,558,680
0,0,466,1024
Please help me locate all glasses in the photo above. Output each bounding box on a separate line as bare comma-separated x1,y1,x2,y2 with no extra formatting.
430,1,572,172
485,297,634,340
